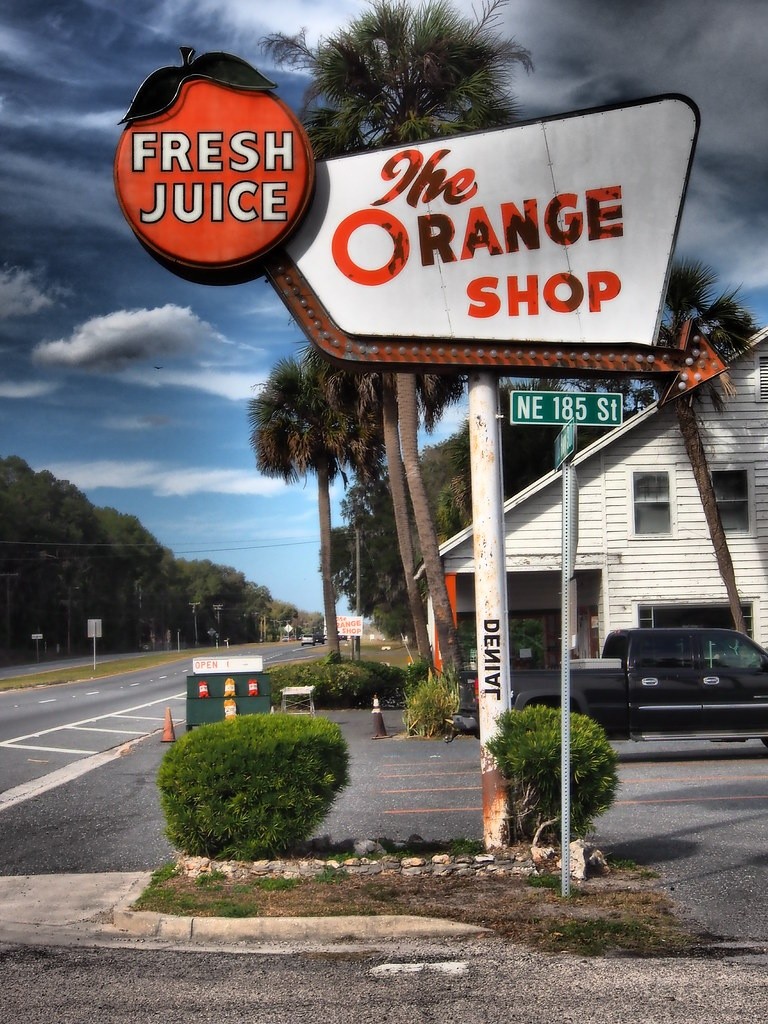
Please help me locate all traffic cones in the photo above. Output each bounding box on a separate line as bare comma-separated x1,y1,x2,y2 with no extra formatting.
160,707,176,742
369,694,393,740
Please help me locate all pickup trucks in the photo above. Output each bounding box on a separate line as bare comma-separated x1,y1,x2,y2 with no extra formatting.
442,625,768,750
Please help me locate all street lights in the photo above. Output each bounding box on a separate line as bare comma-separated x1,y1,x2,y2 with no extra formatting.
212,604,223,622
189,601,201,641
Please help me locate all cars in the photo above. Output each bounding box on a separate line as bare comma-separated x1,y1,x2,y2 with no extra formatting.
300,633,315,646
314,633,325,644
